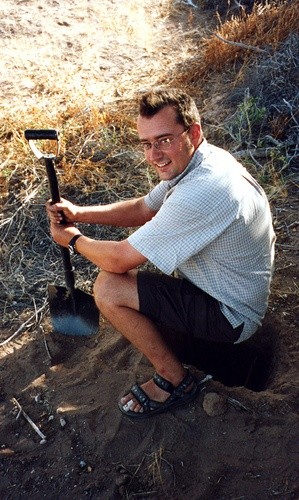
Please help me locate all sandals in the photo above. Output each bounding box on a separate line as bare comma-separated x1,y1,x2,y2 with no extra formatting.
116,372,199,419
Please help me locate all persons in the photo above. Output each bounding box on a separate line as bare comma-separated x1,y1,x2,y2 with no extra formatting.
45,88,276,416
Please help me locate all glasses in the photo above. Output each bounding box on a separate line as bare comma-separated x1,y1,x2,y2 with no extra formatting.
137,130,189,153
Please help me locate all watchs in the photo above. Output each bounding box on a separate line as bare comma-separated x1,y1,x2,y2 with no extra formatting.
68,234,82,255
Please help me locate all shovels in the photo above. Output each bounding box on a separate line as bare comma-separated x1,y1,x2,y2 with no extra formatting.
24,129,99,339
